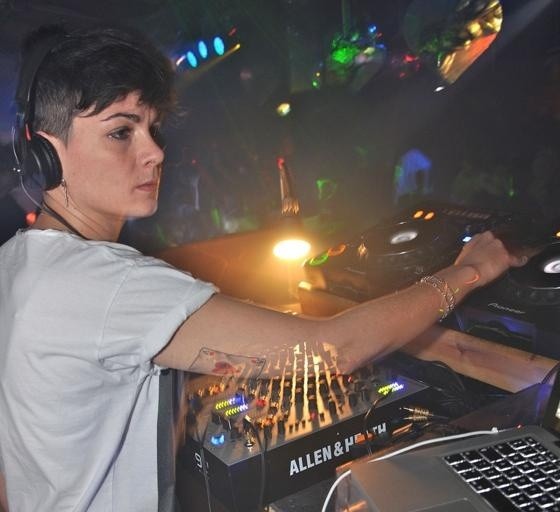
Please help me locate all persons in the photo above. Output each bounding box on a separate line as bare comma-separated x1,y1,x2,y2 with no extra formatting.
0,34,528,512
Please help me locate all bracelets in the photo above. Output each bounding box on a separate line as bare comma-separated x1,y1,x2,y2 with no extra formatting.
421,274,456,323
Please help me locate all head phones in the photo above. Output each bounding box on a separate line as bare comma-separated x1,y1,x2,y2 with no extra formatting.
12,34,89,192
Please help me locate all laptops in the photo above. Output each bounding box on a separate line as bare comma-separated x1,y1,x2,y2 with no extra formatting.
343,365,560,508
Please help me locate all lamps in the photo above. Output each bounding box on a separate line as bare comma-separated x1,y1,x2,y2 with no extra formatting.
272,158,315,259
